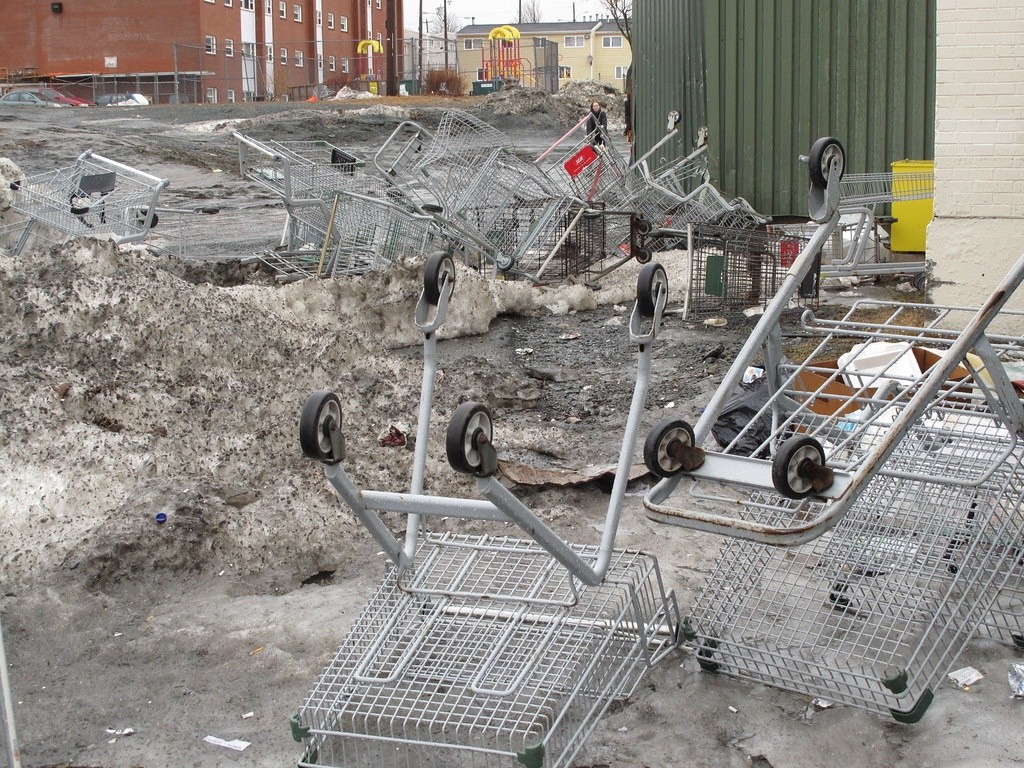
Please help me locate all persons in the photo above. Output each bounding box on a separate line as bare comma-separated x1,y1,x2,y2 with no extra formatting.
586,102,608,147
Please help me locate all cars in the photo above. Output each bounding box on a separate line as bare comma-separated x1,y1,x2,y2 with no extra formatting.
87,92,151,107
0,90,73,109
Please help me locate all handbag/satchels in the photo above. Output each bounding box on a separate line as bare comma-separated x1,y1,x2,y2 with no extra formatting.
589,132,596,144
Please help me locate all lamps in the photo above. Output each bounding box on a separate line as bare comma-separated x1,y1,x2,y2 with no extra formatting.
584,34,590,39
51,2,62,13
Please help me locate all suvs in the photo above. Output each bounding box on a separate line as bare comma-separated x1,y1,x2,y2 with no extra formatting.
9,86,98,108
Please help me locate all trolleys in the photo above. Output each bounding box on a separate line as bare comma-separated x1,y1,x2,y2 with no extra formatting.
635,131,1024,730
7,149,174,260
231,104,932,310
290,246,713,768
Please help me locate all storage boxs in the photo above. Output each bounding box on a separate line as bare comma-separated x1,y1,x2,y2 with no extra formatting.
792,347,973,437
